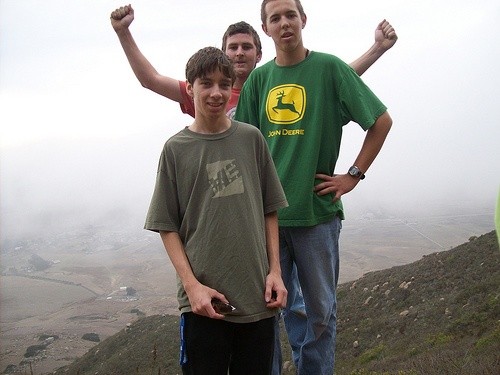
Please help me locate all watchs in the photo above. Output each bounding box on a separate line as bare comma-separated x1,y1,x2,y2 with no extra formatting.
347,166,365,180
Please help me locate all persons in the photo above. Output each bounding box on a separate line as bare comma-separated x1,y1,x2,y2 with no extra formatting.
144,46,288,375
234,0,392,375
110,4,398,121
